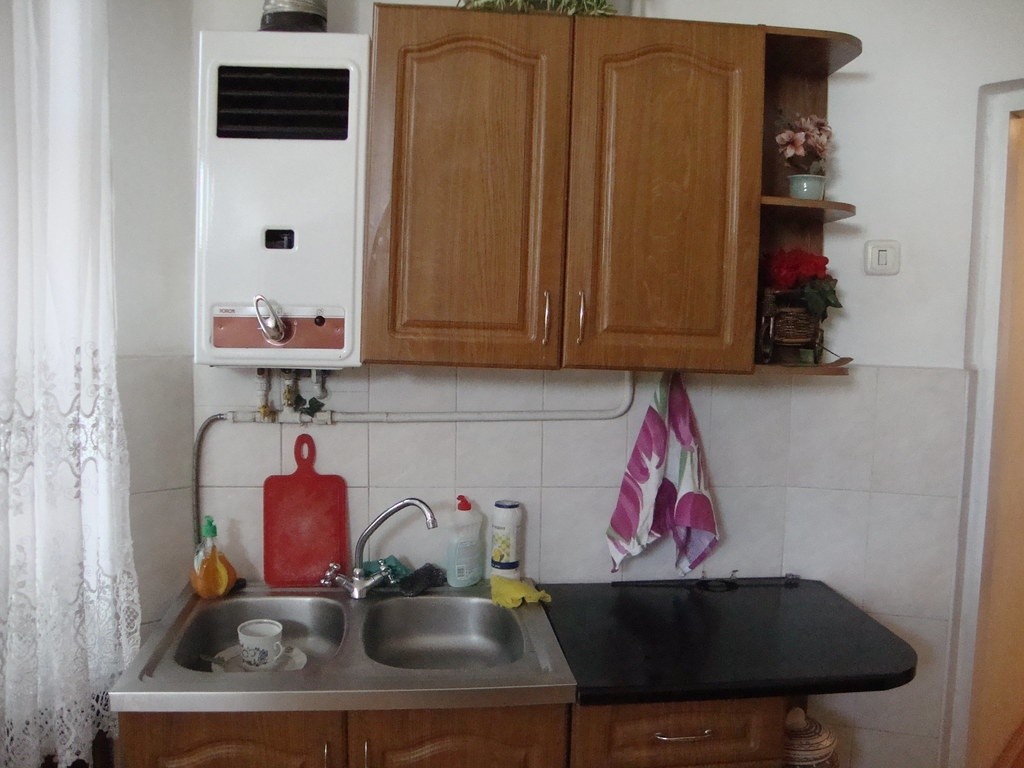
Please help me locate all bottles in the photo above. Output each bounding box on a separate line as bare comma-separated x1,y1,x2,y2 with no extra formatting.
446,496,485,587
492,499,523,581
238,619,283,672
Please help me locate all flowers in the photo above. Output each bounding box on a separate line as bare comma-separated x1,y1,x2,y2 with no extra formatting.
776,109,834,174
768,249,844,321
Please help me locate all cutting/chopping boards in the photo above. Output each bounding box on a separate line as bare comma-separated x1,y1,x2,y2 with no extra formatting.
262,433,350,587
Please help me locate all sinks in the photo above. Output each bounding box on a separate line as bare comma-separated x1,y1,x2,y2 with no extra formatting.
339,577,577,680
134,579,364,686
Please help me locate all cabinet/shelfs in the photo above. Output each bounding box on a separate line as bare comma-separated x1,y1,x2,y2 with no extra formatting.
112,706,568,768
754,24,863,376
355,2,770,376
571,698,793,768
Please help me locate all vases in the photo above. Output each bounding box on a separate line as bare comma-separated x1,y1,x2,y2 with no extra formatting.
770,299,825,345
786,171,828,201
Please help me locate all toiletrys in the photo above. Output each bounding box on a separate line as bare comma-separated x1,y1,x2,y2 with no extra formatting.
193,515,225,600
445,493,485,587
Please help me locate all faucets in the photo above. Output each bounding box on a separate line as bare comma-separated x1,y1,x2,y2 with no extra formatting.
350,497,438,600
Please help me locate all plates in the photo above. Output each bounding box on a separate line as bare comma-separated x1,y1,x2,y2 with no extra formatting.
212,644,307,672
